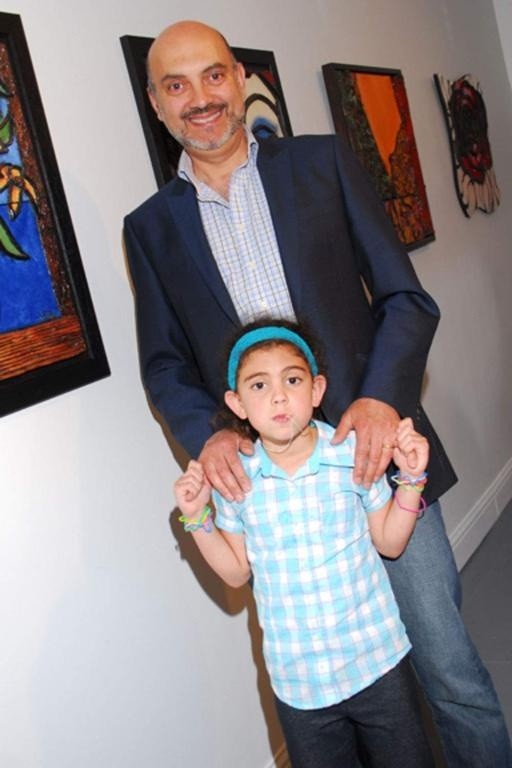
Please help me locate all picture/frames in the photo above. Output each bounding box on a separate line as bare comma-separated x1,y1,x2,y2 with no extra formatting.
322,63,435,253
120,35,293,189
0,9,110,421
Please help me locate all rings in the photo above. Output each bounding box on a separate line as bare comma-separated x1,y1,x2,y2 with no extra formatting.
382,443,395,451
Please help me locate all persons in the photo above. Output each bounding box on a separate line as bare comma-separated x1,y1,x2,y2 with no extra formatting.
119,19,510,765
171,316,434,767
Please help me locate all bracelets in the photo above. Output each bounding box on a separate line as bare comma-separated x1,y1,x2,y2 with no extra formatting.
178,505,215,535
390,470,428,516
